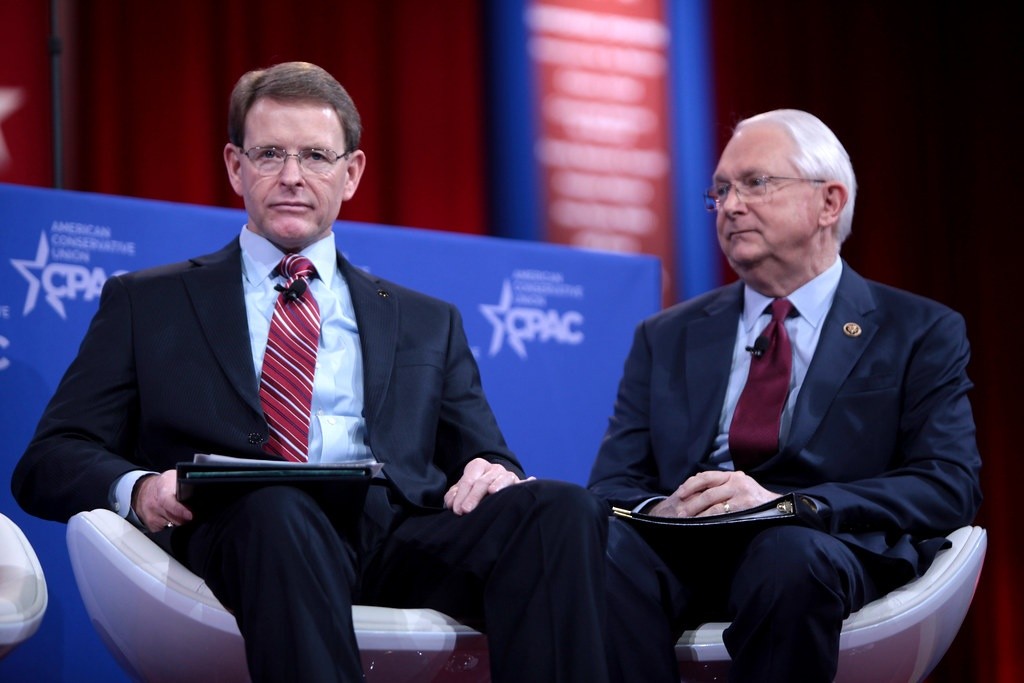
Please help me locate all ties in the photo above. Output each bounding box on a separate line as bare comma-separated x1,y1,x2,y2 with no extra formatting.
259,253,320,463
728,300,800,477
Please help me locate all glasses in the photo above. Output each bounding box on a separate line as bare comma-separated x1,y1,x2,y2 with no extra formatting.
241,147,347,176
704,174,826,211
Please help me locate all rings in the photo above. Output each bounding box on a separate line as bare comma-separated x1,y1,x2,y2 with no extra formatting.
722,499,731,512
167,521,173,527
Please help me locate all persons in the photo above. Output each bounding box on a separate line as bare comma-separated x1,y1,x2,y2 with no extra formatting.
10,60,610,682
587,108,985,683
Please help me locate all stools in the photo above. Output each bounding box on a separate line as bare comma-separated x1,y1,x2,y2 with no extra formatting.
0,512,49,661
65,508,492,683
674,525,988,683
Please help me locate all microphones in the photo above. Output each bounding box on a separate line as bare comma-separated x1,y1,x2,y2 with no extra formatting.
751,336,768,359
287,279,308,301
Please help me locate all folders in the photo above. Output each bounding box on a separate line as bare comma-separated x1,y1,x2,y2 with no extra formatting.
176,460,372,527
610,492,825,532
